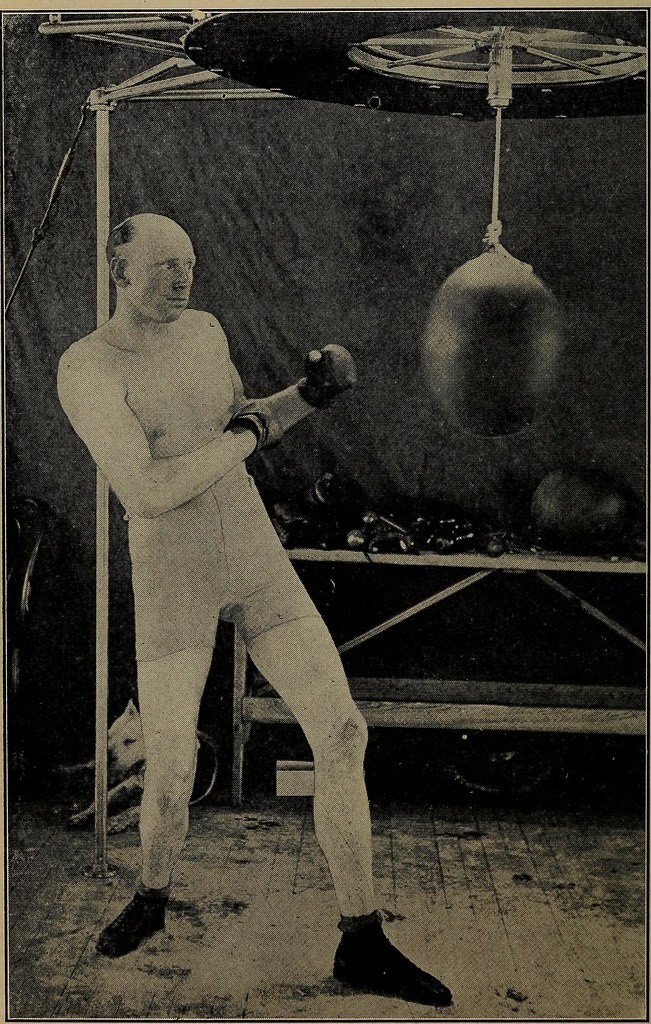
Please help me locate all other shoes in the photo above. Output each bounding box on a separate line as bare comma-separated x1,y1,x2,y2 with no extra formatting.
332,909,453,1007
96,876,174,959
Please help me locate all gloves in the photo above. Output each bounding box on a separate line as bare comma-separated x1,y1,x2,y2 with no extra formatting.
297,343,355,410
223,401,270,453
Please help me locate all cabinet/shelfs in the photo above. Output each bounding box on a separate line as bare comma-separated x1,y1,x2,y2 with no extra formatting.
231,547,651,809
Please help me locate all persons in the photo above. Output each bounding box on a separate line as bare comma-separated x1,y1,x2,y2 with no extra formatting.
56,214,453,1006
